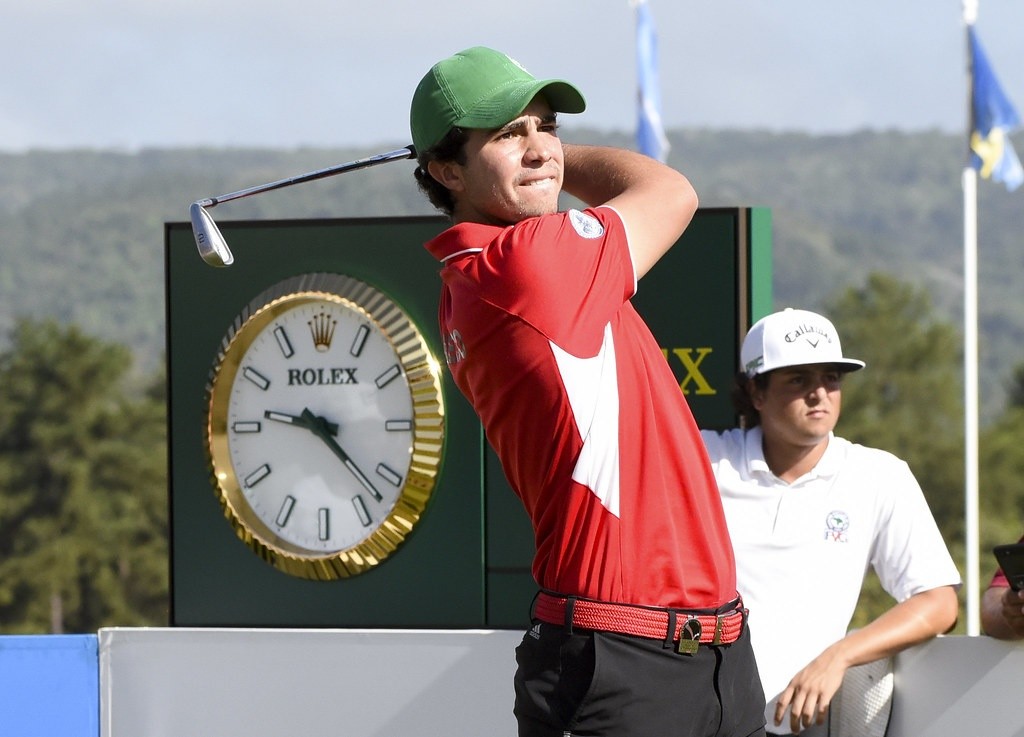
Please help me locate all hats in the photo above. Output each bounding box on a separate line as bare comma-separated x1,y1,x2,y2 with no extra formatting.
410,47,586,163
738,308,868,375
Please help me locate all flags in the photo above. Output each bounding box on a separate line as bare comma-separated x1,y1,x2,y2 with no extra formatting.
963,24,1023,193
635,2,671,165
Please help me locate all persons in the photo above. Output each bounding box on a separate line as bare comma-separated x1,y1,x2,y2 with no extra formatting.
981,527,1024,640
411,46,766,736
698,308,963,736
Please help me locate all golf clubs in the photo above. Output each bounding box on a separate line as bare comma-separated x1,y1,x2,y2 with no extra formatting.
188,142,418,266
